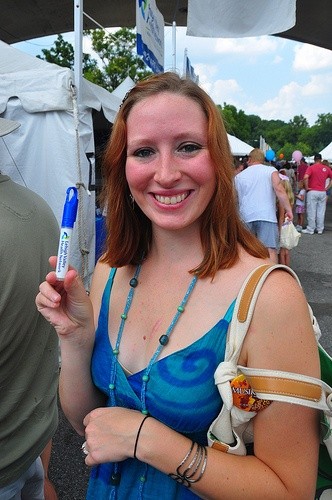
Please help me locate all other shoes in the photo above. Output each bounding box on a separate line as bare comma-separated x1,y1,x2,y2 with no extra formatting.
300,228,315,235
317,230,323,235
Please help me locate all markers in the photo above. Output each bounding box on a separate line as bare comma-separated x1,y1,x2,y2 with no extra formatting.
55,187,79,280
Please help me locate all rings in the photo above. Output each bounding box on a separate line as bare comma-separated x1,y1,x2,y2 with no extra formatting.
82,442,89,454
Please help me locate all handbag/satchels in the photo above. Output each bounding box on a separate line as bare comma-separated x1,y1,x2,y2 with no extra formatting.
202,262,332,464
279,216,302,250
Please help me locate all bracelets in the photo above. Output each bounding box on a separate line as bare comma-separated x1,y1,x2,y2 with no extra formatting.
168,442,207,488
133,415,151,460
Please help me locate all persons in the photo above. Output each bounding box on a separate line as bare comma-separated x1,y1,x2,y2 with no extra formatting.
232,149,332,265
0,117,61,500
35,72,321,500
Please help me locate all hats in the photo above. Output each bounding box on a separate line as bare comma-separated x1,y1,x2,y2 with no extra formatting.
313,154,323,161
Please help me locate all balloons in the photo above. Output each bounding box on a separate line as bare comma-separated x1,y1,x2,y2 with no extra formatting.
266,150,275,160
293,150,302,162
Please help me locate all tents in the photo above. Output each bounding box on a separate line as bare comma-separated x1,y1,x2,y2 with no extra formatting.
113,74,254,156
0,41,120,291
306,141,332,163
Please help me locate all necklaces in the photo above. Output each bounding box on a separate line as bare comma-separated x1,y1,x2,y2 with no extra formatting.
109,264,198,500
